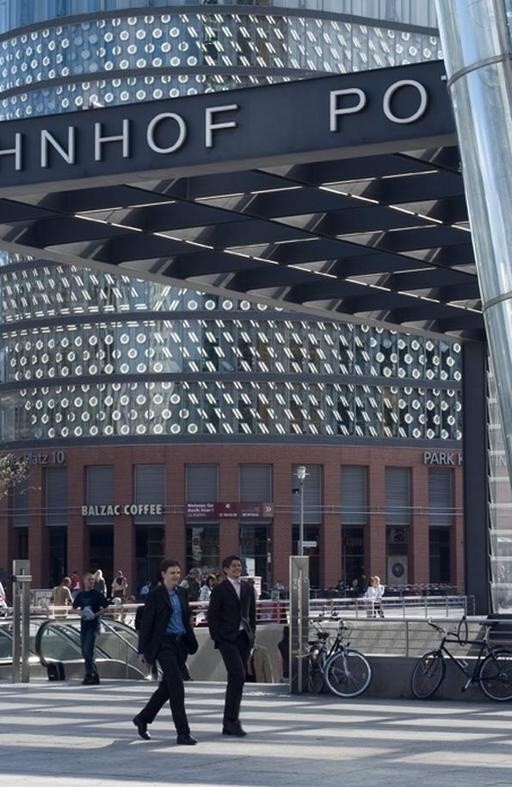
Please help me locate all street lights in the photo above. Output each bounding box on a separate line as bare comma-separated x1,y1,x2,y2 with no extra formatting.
292,463,310,555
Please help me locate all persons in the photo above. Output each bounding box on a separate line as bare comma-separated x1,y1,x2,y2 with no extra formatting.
111,568,128,599
335,573,384,618
139,581,151,600
180,565,217,626
130,558,199,746
49,576,74,618
70,571,80,592
93,569,106,597
72,573,110,685
207,555,257,738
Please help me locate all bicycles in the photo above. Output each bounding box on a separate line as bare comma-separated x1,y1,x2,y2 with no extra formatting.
411,612,512,705
304,616,374,698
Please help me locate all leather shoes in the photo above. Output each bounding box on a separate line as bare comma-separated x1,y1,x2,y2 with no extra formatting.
133,715,151,739
177,733,197,745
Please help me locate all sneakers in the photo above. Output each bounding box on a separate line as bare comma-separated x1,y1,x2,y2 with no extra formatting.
222,718,246,736
82,676,99,685
